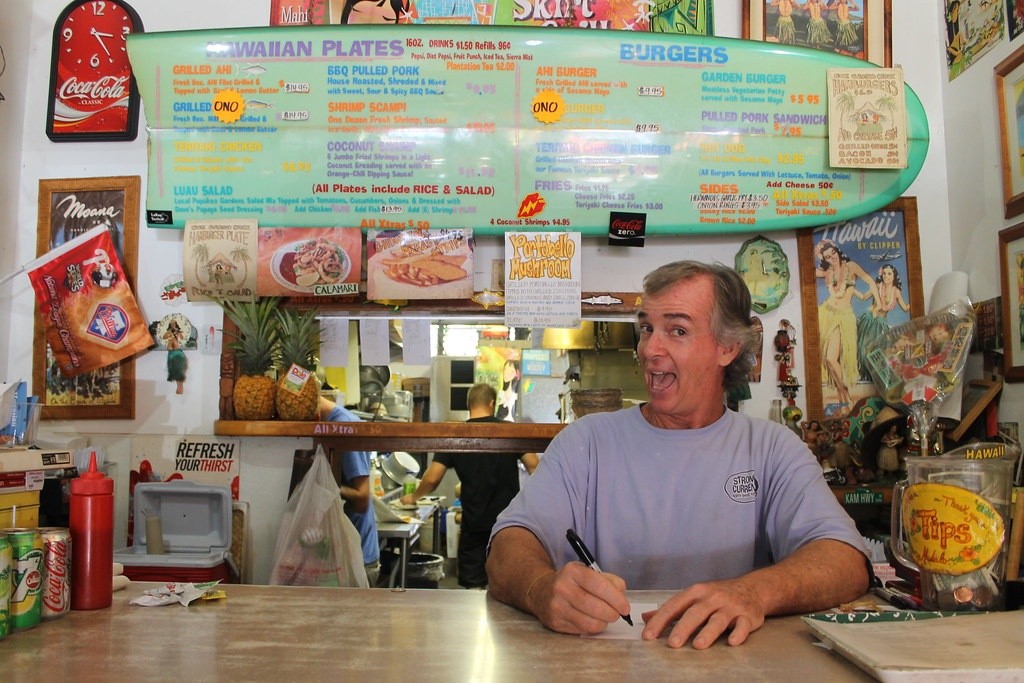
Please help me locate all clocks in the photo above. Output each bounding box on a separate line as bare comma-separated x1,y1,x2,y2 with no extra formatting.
46,0,145,141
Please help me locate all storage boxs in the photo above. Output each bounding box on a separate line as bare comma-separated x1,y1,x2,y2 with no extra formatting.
113,479,240,583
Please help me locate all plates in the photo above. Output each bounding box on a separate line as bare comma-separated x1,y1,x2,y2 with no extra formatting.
270,240,351,294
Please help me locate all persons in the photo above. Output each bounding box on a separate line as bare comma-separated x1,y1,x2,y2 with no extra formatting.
401,383,539,590
484,260,875,650
316,396,381,588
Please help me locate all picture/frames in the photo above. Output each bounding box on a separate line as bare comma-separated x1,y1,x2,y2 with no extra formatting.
998,222,1024,383
993,44,1024,219
795,197,926,422
742,0,892,68
33,175,142,421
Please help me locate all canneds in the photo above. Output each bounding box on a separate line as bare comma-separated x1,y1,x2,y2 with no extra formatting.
0,526,72,640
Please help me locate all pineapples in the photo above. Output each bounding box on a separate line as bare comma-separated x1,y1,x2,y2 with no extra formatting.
209,291,325,422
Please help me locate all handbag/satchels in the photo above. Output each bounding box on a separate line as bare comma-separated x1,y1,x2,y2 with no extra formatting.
263,443,368,588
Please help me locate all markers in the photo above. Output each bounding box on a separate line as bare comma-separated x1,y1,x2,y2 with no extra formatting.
565,528,633,626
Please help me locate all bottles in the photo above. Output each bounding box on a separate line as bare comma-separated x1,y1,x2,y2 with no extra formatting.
403,471,416,504
369,460,385,497
67,452,114,612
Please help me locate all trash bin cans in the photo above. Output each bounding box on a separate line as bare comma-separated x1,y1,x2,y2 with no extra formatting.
392,552,445,589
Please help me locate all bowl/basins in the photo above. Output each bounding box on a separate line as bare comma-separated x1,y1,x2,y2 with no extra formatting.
3,402,44,447
380,452,421,486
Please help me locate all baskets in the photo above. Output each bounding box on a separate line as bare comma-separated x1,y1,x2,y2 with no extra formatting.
568,388,625,417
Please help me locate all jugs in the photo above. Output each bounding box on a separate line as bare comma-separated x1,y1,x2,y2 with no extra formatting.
890,441,1024,614
